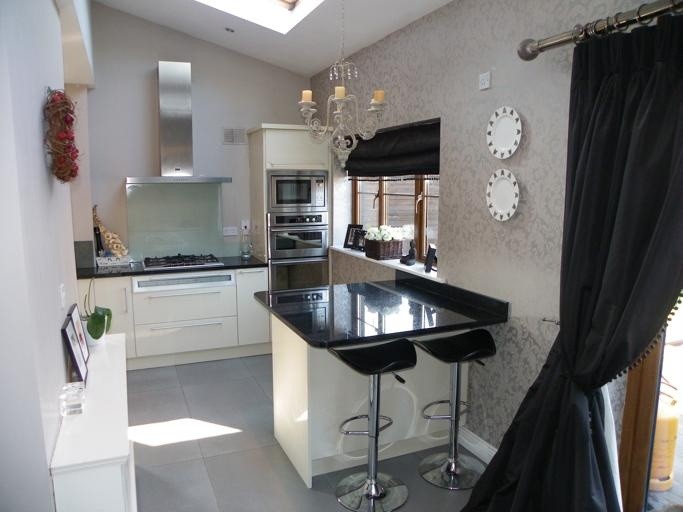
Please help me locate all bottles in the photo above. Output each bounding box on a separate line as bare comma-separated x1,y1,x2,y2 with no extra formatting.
94,227,105,257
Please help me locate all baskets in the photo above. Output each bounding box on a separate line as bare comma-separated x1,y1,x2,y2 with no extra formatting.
363,238,403,261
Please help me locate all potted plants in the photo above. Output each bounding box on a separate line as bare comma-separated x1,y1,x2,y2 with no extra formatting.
79,278,112,346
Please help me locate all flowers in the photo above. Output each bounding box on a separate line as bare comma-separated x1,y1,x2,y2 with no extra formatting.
365,225,402,241
44,87,78,183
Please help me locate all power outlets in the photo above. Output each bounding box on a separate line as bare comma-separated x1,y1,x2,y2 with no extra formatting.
241,219,251,234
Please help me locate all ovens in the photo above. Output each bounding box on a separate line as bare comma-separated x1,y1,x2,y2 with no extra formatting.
270,302,330,343
265,226,329,289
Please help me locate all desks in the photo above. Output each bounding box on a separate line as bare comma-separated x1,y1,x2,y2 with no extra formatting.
51,333,138,512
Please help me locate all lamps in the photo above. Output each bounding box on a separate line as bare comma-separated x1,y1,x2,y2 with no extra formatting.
298,0,385,168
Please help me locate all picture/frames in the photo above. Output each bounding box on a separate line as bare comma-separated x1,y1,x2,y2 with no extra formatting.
61,316,88,386
352,230,367,251
425,244,437,272
67,303,90,362
344,224,363,247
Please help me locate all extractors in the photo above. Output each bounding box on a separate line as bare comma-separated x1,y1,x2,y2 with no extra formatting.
124,60,233,185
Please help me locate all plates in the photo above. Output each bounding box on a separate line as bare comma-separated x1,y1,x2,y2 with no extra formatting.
483,169,520,221
485,107,525,160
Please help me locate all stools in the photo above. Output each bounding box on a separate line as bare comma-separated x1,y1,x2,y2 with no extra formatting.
327,338,416,512
413,329,496,491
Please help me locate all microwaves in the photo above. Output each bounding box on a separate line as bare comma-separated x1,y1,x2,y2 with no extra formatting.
271,176,326,207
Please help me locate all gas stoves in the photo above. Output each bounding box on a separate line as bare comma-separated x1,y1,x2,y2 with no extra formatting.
142,253,223,272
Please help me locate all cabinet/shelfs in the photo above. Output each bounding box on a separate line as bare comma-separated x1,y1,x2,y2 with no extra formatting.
78,277,137,371
236,267,270,357
247,123,332,170
